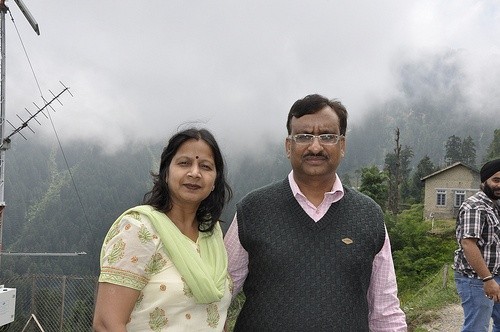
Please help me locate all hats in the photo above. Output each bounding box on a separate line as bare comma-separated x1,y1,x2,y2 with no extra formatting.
480,159,500,183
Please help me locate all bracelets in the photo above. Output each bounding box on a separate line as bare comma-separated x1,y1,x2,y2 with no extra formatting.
481,276,494,282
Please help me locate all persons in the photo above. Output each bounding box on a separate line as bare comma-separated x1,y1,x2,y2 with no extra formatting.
90,130,232,332
454,158,500,332
221,94,413,331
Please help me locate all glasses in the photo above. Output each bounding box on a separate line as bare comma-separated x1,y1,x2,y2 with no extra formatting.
287,134,345,145
